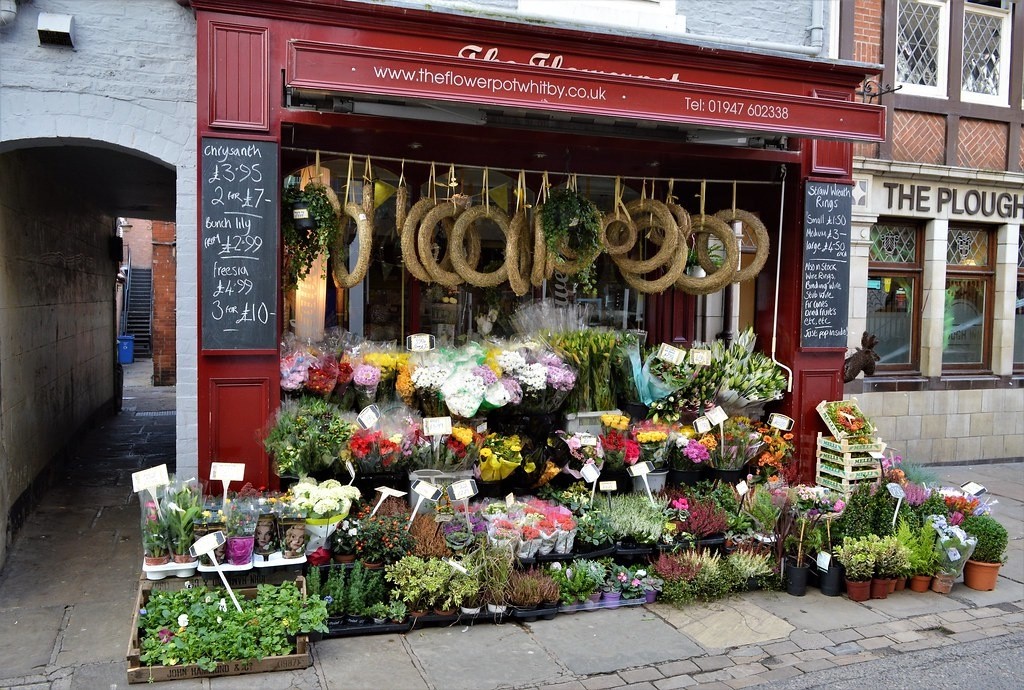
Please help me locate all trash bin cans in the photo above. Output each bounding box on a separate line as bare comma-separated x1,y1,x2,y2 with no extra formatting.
117,335,135,363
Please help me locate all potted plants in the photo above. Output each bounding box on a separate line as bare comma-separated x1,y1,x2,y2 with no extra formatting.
386,600,410,625
531,567,560,610
367,601,391,625
551,520,1009,613
511,570,542,611
323,578,347,629
348,576,369,627
433,559,478,616
479,542,516,613
384,554,450,617
457,544,499,614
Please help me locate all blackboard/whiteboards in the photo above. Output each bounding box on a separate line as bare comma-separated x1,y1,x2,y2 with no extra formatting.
199,134,282,356
799,178,853,351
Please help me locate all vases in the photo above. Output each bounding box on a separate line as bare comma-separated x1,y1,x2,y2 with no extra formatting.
144,552,169,566
226,536,254,565
173,552,198,563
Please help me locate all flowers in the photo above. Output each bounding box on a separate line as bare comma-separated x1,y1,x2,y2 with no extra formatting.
168,482,201,556
222,499,256,536
193,505,227,526
142,498,171,559
255,332,985,547
